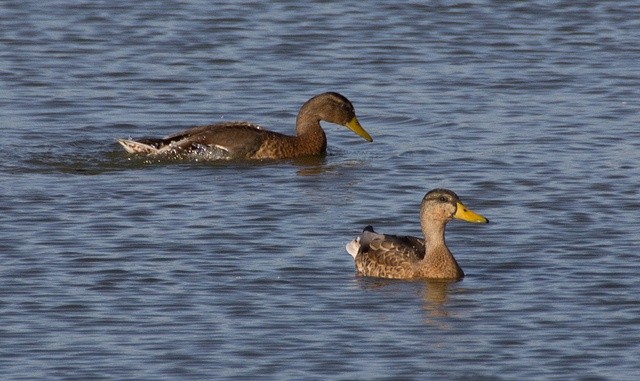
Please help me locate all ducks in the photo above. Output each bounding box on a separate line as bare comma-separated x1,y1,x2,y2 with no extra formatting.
113,91,374,162
344,188,491,282
